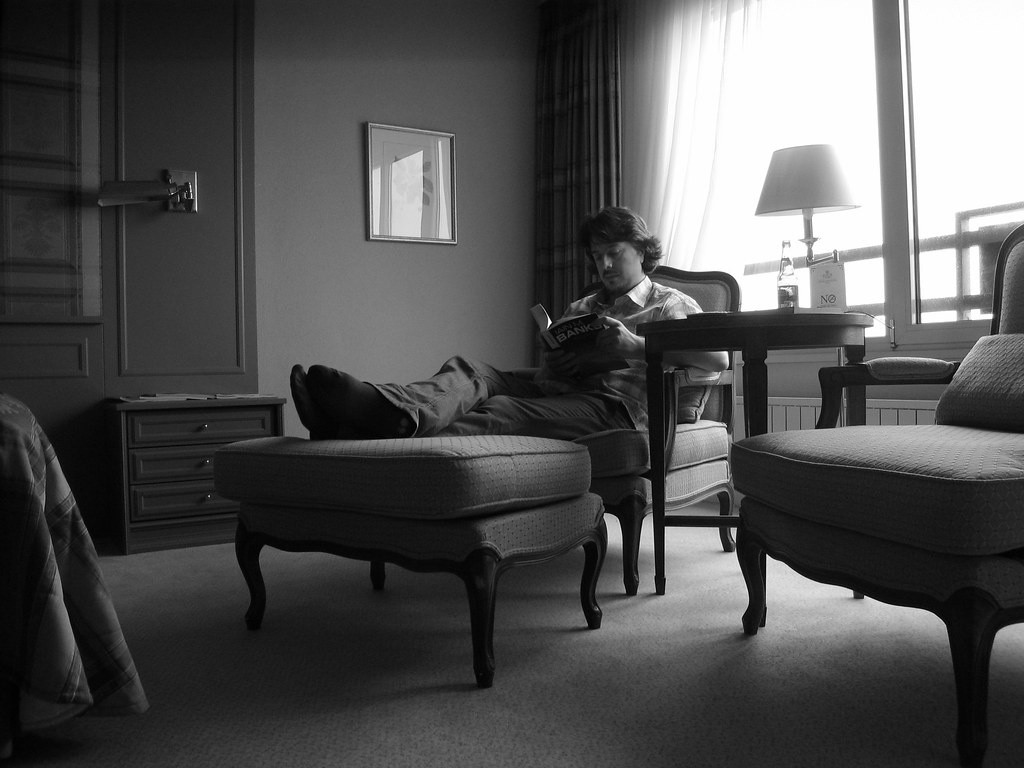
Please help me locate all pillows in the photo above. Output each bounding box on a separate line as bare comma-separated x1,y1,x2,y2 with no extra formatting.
675,384,712,424
933,333,1024,436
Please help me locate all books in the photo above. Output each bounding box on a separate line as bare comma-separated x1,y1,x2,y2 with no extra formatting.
686,305,844,314
528,304,630,378
119,393,277,401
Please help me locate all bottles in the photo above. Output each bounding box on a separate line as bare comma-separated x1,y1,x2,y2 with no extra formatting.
775,240,800,308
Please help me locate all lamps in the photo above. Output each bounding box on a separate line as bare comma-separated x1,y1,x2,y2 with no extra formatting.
754,144,861,264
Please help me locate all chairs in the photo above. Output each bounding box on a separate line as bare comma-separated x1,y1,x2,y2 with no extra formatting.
731,216,1024,768
563,265,740,598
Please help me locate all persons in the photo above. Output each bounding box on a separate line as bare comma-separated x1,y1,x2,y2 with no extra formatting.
289,204,707,439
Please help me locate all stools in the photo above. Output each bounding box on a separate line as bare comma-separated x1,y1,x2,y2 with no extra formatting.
219,436,608,687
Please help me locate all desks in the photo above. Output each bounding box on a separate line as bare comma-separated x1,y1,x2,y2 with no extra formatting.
634,309,874,626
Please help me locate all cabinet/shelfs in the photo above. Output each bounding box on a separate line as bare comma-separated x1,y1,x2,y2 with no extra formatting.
106,394,286,555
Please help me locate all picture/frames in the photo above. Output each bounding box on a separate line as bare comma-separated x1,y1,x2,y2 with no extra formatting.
364,121,458,245
